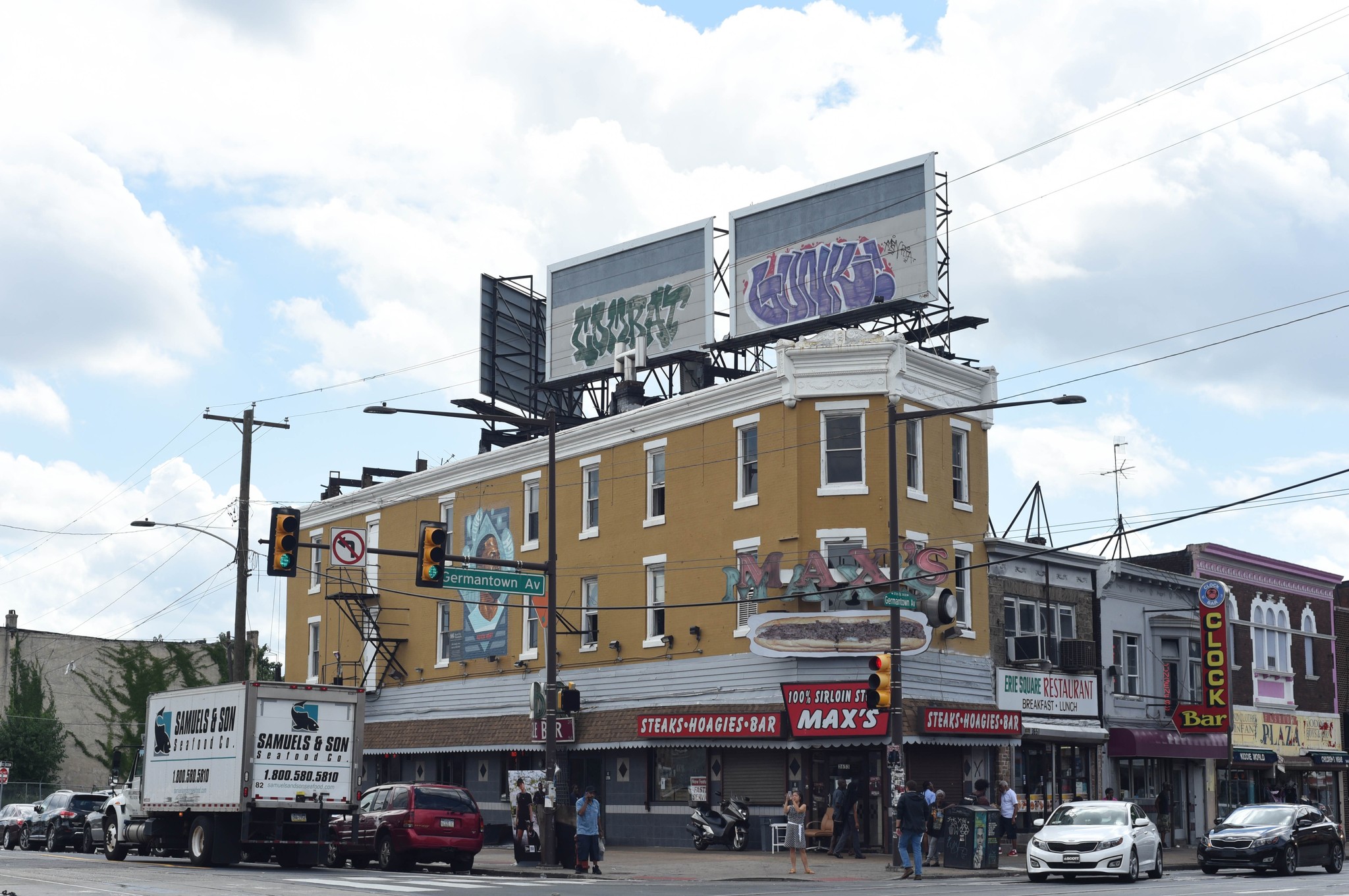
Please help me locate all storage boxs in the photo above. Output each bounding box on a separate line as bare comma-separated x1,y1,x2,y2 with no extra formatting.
688,800,706,807
658,777,690,801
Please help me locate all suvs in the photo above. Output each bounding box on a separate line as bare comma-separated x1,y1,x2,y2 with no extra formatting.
323,781,485,872
19,790,112,853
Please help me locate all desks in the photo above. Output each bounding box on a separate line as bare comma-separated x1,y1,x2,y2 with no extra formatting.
770,823,788,854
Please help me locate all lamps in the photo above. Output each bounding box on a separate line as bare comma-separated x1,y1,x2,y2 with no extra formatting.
487,655,499,663
942,627,963,640
513,660,528,668
689,625,700,640
458,661,467,666
608,640,620,652
1109,665,1123,677
943,335,949,348
874,296,884,304
722,334,731,340
387,669,402,680
661,635,672,648
414,667,423,672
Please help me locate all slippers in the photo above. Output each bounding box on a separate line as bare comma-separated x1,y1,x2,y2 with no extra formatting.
789,869,796,873
804,869,815,874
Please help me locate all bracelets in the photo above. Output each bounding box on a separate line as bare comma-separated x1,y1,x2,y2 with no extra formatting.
792,800,794,802
896,827,900,829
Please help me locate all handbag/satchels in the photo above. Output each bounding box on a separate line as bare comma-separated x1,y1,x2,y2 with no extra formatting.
598,837,605,861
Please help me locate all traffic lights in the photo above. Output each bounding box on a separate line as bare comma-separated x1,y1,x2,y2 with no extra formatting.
415,520,449,590
266,508,301,579
557,688,580,713
867,654,891,709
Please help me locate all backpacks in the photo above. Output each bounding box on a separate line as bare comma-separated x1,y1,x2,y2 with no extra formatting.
958,792,984,805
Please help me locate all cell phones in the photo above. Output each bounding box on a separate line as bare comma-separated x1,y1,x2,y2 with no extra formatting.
585,792,590,799
788,791,792,798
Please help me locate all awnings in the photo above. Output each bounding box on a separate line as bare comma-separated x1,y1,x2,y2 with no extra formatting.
1107,727,1228,759
1232,746,1277,763
1299,748,1349,765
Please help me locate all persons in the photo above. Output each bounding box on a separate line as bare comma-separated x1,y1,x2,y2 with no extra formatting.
922,790,955,867
996,780,1018,856
1155,782,1173,849
827,778,866,859
1103,788,1118,801
783,791,815,874
920,781,936,859
896,780,930,880
575,786,602,875
1300,795,1312,806
567,784,580,806
515,778,545,848
970,779,990,807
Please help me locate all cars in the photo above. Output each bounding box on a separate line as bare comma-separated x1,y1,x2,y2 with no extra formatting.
81,792,152,856
0,803,43,850
1197,803,1345,877
1026,800,1164,884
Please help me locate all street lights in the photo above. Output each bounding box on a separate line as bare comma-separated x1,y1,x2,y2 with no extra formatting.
363,401,562,868
888,393,1087,869
130,520,249,684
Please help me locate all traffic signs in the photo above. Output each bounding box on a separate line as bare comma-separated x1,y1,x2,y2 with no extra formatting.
0,768,9,784
0,761,13,769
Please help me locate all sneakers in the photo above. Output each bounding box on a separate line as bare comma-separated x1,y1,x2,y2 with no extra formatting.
592,865,602,874
999,848,1002,854
1162,842,1171,849
575,865,582,874
1007,849,1018,856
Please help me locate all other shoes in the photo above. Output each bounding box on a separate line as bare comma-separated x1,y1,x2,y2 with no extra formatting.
931,864,940,867
922,862,930,867
827,852,834,856
855,854,866,859
833,852,843,858
848,852,855,855
901,868,914,879
914,874,922,880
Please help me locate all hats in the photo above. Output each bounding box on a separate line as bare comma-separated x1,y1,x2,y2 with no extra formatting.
517,778,524,787
585,785,596,792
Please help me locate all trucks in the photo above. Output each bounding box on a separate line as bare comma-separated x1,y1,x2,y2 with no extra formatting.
101,679,367,868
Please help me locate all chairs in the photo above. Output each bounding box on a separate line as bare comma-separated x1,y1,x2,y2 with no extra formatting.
804,807,834,852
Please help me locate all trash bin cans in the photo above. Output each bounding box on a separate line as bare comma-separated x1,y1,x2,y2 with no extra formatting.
943,805,988,870
759,815,788,851
972,805,1000,869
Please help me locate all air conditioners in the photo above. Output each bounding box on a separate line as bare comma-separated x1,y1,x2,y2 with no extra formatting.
1008,635,1046,662
1061,640,1096,666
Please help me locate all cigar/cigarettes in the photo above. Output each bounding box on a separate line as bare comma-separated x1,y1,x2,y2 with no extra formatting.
858,829,859,832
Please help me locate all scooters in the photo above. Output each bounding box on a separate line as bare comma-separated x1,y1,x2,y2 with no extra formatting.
685,791,751,851
